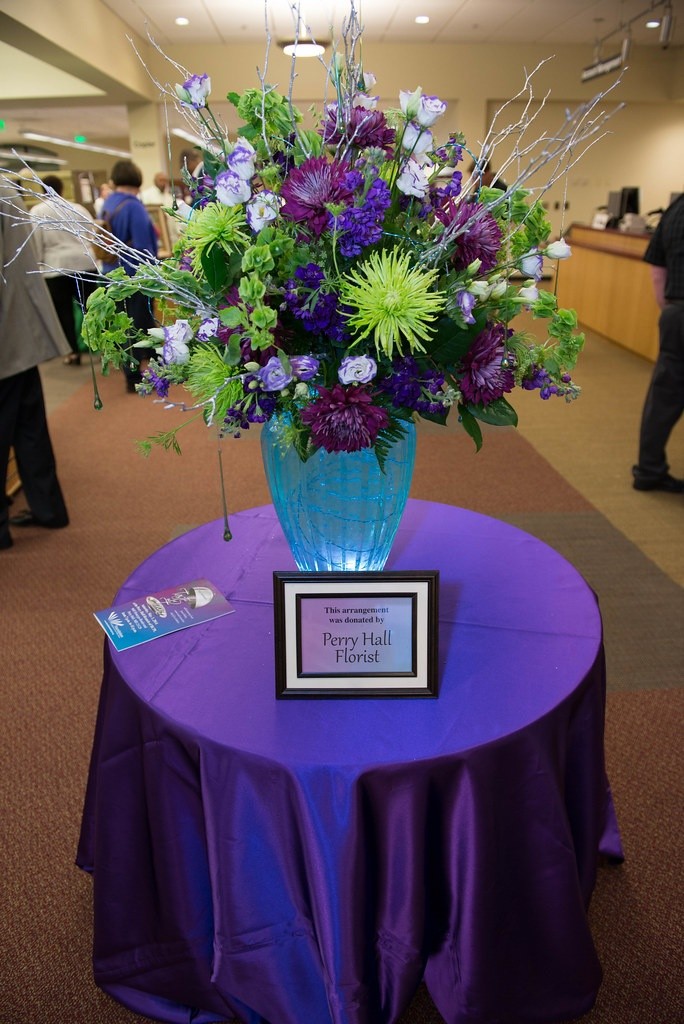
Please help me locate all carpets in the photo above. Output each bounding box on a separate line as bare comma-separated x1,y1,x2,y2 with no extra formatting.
0,359,683,1024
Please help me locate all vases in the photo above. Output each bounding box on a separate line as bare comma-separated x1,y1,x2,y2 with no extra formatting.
259,383,417,571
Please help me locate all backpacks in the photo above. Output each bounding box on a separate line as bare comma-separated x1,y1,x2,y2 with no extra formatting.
86,199,131,265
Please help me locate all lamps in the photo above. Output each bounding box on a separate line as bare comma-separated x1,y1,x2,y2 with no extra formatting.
660,0,675,49
621,22,632,62
279,41,328,58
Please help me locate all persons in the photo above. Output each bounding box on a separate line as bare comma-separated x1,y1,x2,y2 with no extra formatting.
94,160,161,394
0,179,70,549
29,174,118,365
474,156,512,225
633,193,684,494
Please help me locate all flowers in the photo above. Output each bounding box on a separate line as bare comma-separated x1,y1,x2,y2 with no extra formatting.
81,73,586,456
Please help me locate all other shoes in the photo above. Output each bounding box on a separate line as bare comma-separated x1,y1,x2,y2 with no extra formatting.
0,537,12,547
10,514,67,527
633,474,684,493
63,354,80,366
127,383,137,393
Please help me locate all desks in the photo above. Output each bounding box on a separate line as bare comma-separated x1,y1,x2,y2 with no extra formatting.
75,499,624,1024
